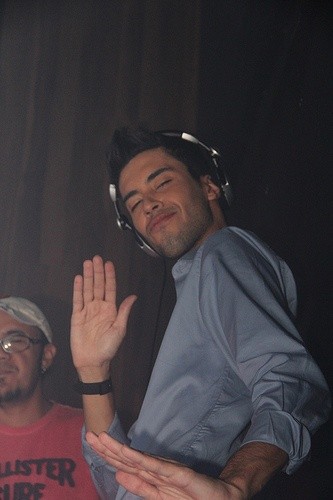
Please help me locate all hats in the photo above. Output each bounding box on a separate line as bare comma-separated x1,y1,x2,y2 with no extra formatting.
0,296,53,344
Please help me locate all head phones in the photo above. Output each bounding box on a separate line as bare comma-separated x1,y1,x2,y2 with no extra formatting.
109,130,234,258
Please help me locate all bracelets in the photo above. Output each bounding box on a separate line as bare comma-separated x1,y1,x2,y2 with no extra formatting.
77,376,113,396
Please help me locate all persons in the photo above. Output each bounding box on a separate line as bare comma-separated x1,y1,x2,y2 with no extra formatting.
68,132,333,500
0,297,104,500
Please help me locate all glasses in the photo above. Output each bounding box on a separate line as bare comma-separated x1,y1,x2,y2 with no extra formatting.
0,332,47,354
